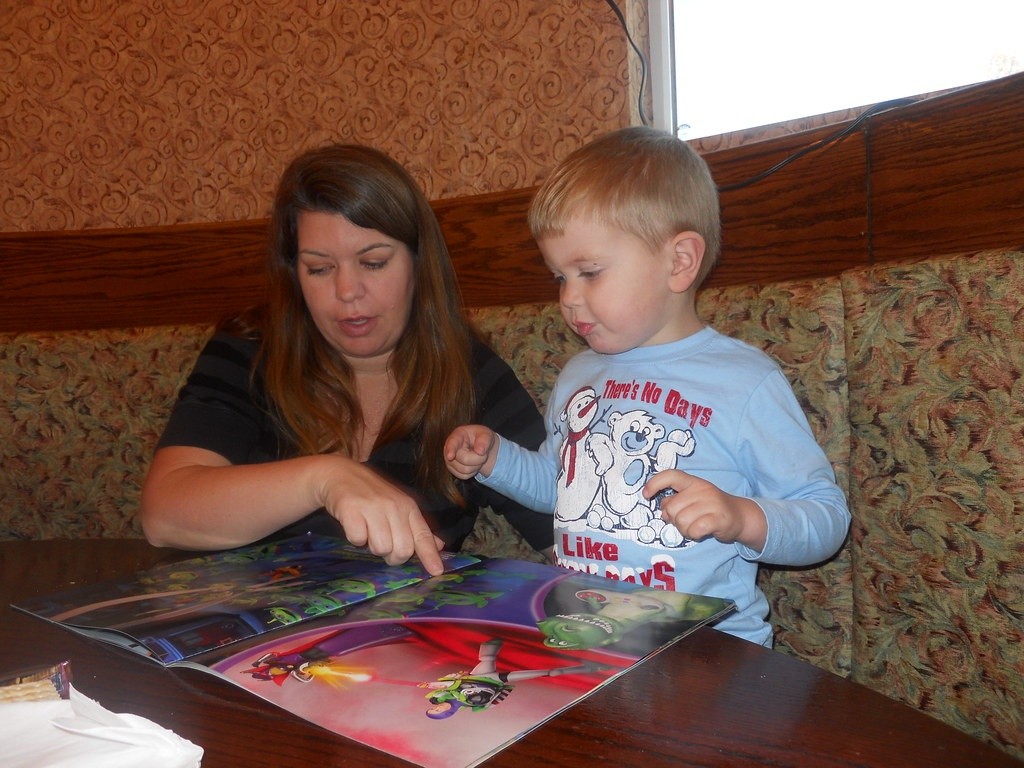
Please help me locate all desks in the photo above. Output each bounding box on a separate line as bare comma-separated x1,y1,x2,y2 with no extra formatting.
1,537,1024,768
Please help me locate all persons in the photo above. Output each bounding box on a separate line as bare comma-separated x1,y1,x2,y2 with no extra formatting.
443,127,852,650
140,142,568,578
